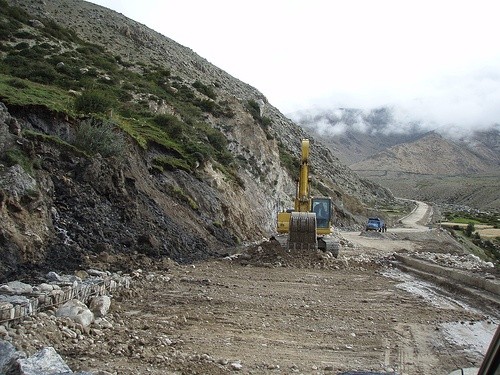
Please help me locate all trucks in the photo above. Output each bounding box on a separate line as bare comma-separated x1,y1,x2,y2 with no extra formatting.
366,218,387,233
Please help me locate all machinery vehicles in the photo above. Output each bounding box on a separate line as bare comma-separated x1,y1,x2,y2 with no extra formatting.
277,138,339,259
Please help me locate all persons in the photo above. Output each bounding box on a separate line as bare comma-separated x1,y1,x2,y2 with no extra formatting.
314,202,326,219
382,223,385,233
385,224,387,232
